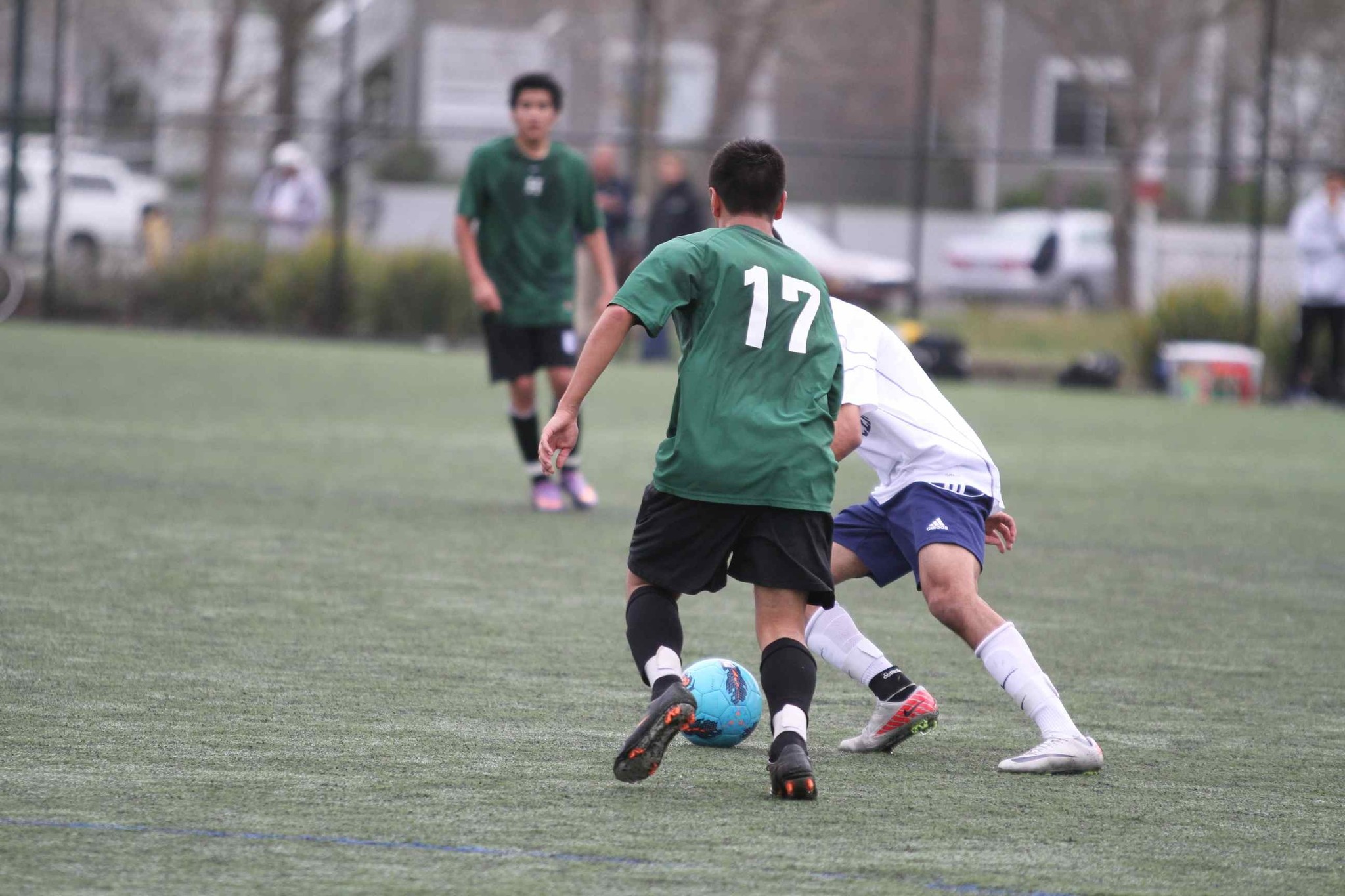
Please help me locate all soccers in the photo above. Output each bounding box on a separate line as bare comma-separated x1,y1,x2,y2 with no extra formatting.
681,657,763,748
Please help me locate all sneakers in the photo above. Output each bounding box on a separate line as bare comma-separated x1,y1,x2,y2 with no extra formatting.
766,743,818,799
839,685,939,753
997,737,1104,774
614,682,698,784
531,479,567,513
560,470,598,511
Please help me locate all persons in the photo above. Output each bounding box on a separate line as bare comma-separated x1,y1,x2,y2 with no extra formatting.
766,226,1108,776
257,142,333,253
455,72,622,513
584,142,640,311
645,142,710,252
532,139,851,800
1283,162,1344,407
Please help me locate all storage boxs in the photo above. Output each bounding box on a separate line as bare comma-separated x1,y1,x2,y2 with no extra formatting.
1158,341,1265,405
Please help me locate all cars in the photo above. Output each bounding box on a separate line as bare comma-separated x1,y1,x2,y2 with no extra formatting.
0,141,174,267
946,204,1152,310
772,214,917,321
